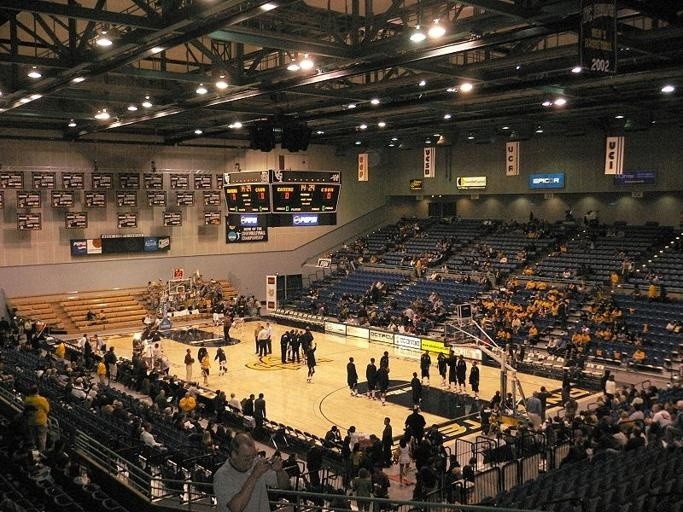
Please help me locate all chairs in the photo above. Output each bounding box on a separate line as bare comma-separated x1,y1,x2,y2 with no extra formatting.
280,215,682,373
463,386,683,512
86,350,222,510
220,402,352,510
1,330,88,511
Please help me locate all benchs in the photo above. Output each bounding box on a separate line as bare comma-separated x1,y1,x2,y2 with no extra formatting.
5,276,241,330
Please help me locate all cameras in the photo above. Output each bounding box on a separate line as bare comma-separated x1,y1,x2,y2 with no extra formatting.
262,459,274,465
